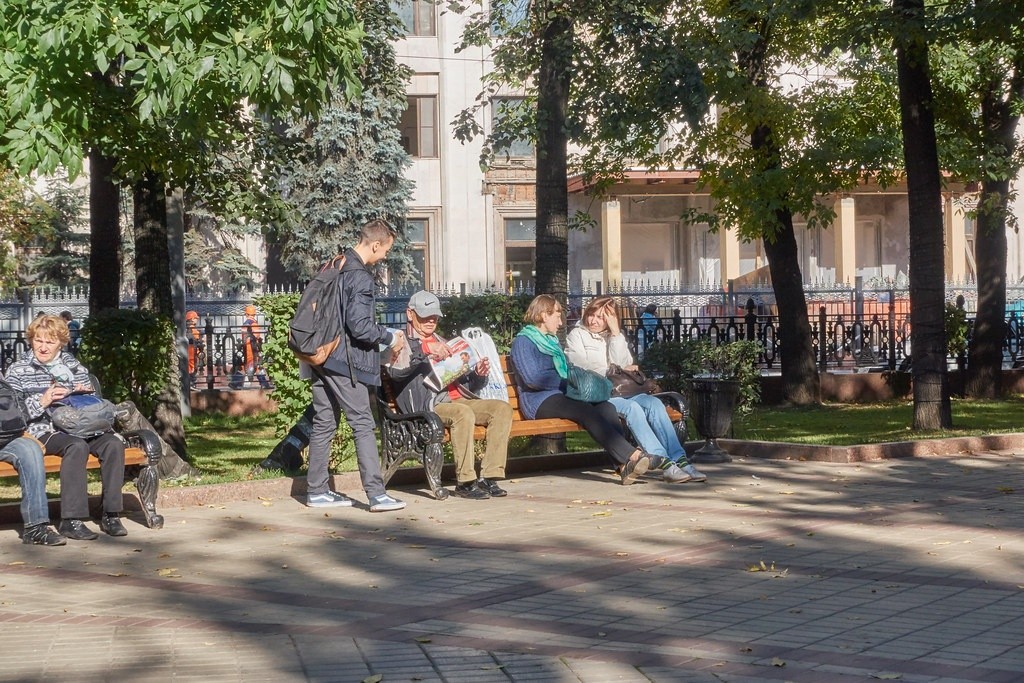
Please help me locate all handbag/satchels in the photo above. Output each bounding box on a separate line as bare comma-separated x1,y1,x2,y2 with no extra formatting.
564,353,613,402
461,327,509,403
607,363,657,398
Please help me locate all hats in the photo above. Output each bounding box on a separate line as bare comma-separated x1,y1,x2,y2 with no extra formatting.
246,306,256,315
408,290,443,318
186,311,200,321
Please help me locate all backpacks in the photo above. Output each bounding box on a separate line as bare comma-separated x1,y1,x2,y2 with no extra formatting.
287,255,373,365
39,387,117,438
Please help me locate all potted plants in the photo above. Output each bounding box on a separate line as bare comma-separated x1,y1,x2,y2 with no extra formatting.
680,337,767,461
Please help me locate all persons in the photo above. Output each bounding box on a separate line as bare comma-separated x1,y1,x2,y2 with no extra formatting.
511,295,661,485
461,353,477,375
639,304,658,350
0,372,67,545
5,315,128,540
563,297,707,482
384,291,513,499
61,312,80,350
307,219,407,510
186,311,199,389
229,307,270,389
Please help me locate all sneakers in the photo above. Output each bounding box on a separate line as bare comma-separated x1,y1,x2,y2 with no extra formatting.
368,493,407,511
306,490,357,506
101,512,128,536
681,464,707,481
22,525,67,545
60,519,99,539
663,463,691,483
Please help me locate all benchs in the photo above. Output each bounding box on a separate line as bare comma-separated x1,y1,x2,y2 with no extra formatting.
380,355,691,499
0,374,164,529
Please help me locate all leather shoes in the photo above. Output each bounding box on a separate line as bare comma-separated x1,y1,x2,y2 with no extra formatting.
621,457,649,485
481,479,507,496
640,452,665,470
456,480,492,498
